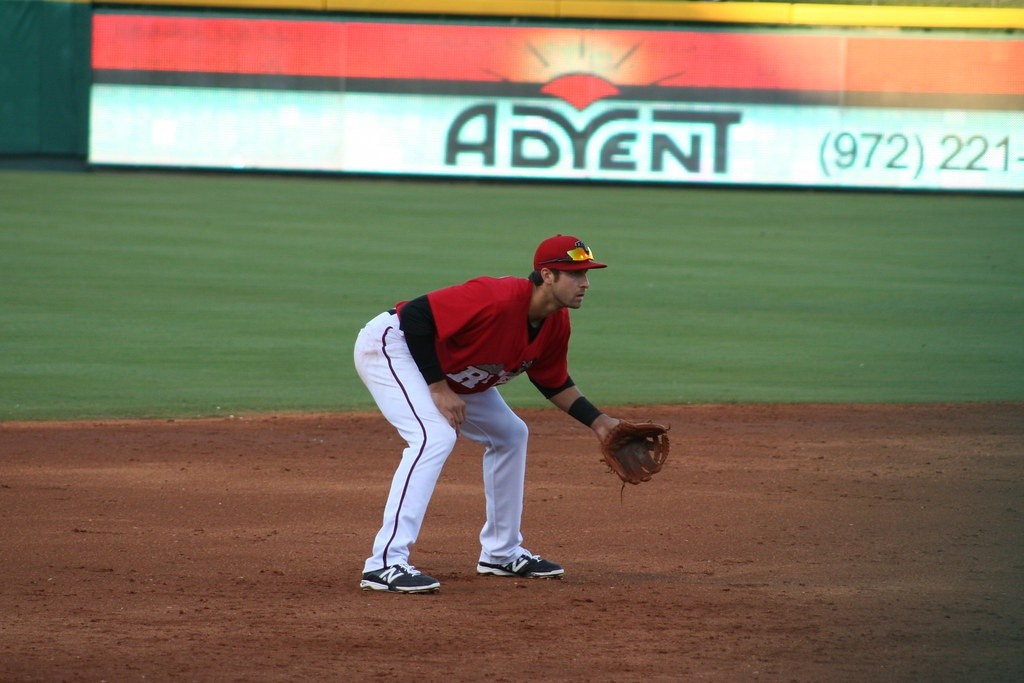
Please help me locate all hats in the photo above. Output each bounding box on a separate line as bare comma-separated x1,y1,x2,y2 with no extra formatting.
534,234,607,271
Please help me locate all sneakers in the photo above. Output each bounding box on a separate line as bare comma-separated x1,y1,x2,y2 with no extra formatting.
360,564,441,594
477,549,564,578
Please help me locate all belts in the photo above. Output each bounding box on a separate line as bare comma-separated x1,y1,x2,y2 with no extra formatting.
387,308,402,331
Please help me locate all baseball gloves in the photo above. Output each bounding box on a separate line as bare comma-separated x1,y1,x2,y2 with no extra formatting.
597,415,673,510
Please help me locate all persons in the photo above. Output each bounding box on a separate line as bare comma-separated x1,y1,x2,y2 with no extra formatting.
353,233,672,594
